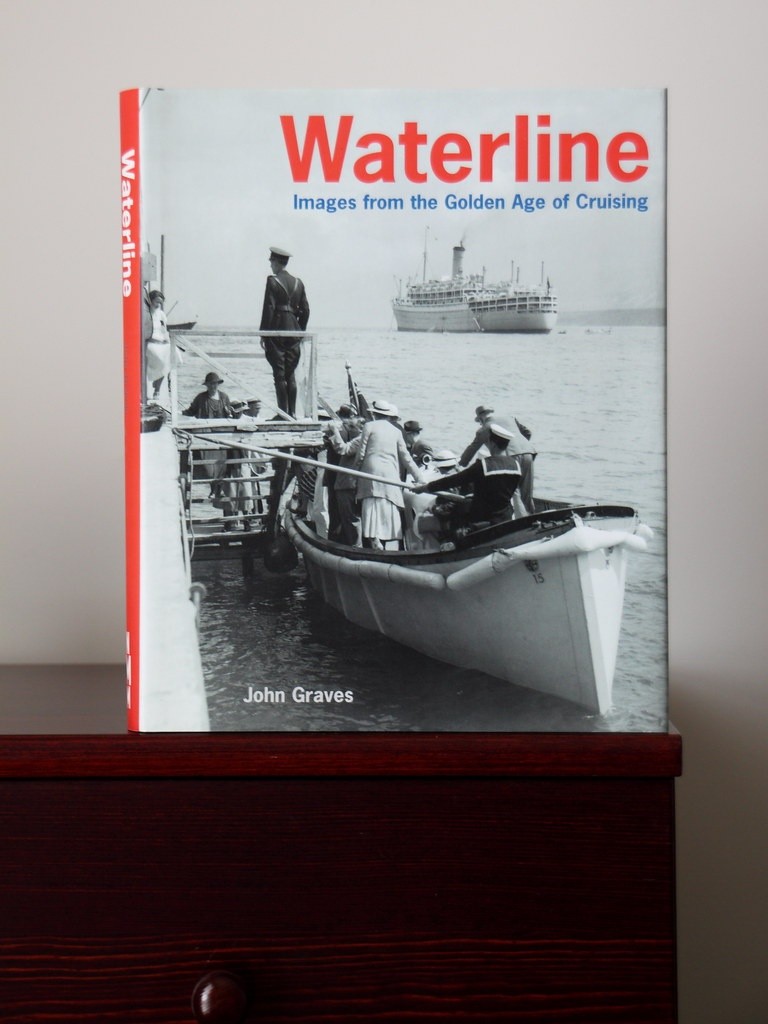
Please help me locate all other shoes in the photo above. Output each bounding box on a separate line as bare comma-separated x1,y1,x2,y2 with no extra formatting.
244,522,250,531
221,527,230,532
289,507,307,517
208,492,215,499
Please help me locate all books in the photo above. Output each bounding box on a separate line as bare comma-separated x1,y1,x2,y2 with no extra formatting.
115,83,670,736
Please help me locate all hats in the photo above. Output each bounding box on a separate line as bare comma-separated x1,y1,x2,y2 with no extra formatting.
246,398,263,408
474,405,494,422
489,424,515,443
366,400,395,417
230,400,249,413
404,421,422,432
389,404,401,421
202,372,223,385
268,247,293,263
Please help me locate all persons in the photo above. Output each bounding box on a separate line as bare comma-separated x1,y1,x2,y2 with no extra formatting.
408,423,526,545
258,248,310,422
457,406,538,516
288,401,463,552
145,289,183,401
177,372,235,500
213,396,263,532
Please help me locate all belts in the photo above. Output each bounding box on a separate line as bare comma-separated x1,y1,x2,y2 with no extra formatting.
276,305,296,315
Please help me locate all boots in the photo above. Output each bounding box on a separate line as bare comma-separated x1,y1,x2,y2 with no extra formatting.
265,381,289,421
288,380,297,420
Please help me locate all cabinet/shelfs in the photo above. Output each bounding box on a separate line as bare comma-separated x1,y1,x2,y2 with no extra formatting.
0,716,683,1024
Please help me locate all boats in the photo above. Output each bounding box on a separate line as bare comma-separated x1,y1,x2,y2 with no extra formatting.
389,225,559,334
276,423,643,716
